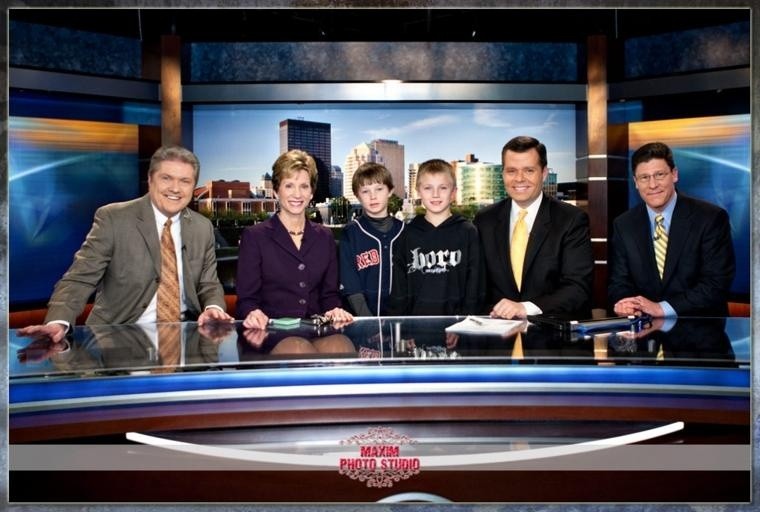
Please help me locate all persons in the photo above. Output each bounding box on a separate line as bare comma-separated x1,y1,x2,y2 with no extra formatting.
16,323,235,380
13,141,237,344
605,318,742,368
390,156,484,351
470,135,596,321
237,322,360,370
392,320,470,360
342,319,393,360
467,322,601,365
337,160,410,351
604,140,737,320
234,146,356,356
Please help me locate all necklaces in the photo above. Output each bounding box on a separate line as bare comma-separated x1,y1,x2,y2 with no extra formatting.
284,228,306,237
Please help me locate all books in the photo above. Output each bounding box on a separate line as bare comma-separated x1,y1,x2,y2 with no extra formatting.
444,313,528,336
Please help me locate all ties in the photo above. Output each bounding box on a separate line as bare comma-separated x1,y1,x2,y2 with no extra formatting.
513,330,524,359
511,209,530,290
656,344,664,360
156,219,182,322
655,215,669,280
158,323,180,372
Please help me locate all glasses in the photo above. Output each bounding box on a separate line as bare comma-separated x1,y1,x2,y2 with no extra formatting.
635,171,671,183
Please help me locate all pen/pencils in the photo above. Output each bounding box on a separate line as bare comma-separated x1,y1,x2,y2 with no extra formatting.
469,318,483,327
